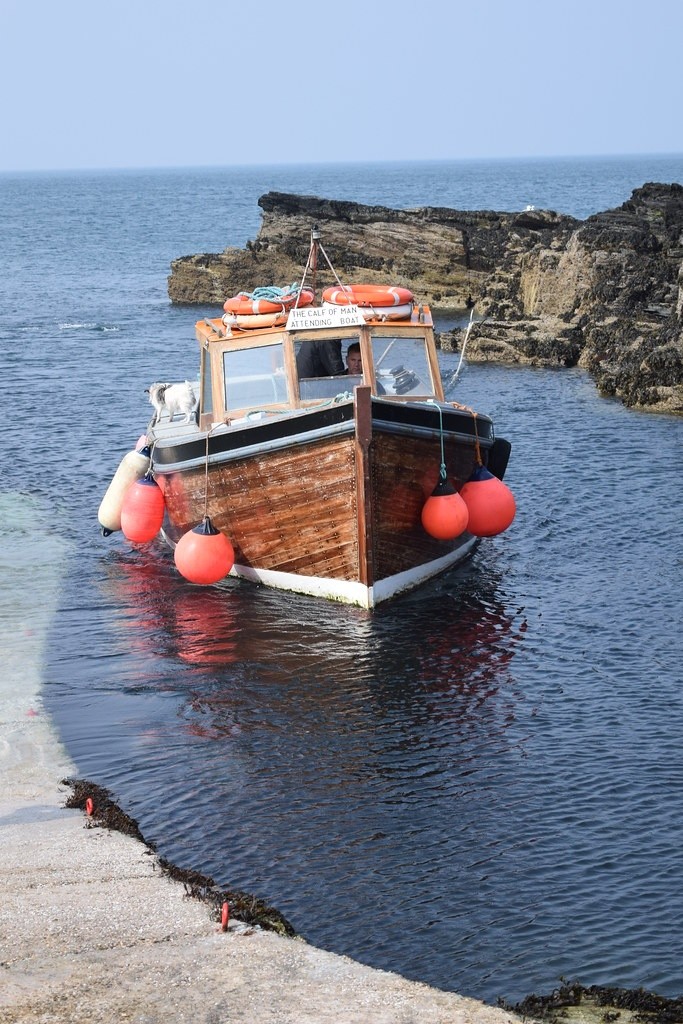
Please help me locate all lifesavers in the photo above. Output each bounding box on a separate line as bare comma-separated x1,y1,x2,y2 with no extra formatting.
322,284,415,321
222,285,315,329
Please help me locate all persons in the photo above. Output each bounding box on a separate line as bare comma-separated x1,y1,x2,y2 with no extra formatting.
296,338,344,378
326,341,386,394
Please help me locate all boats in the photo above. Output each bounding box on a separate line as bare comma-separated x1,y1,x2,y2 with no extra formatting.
94,224,519,617
